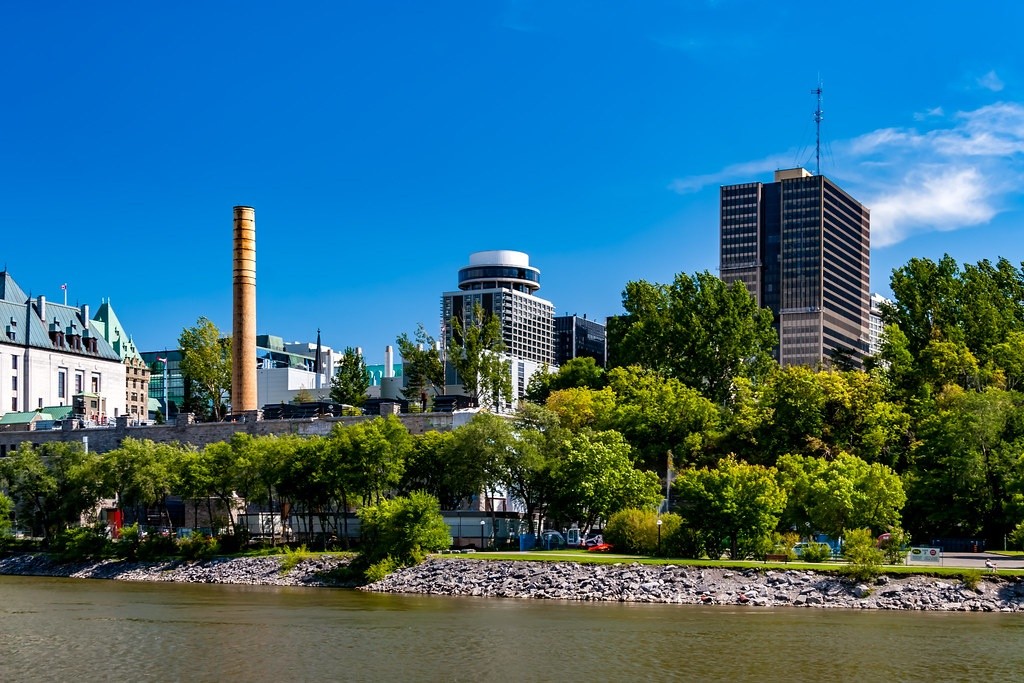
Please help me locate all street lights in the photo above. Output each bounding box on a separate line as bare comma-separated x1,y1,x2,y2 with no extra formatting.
657,519,663,557
480,520,485,551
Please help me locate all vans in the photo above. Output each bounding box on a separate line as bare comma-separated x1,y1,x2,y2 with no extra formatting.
790,541,833,560
